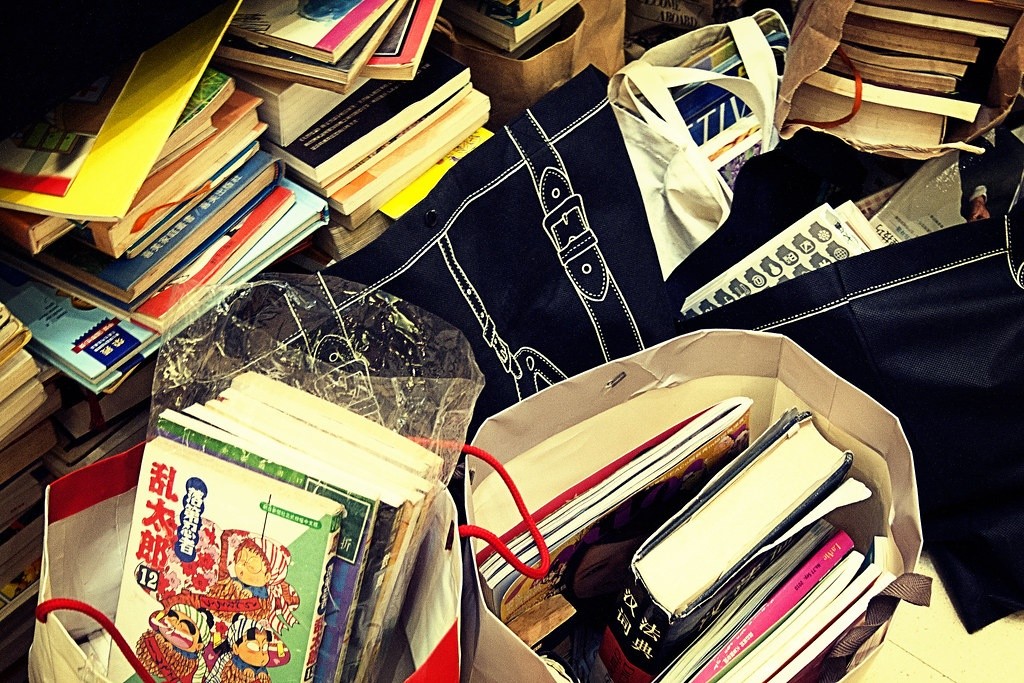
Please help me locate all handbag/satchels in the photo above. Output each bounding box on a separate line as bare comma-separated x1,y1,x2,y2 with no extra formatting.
607,7,799,282
462,330,932,678
27,434,552,683
432,1,586,128
774,2,1023,160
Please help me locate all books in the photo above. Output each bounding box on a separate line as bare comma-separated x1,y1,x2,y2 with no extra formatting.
0,301,62,682
2,0,494,477
75,370,444,682
442,1,578,58
476,396,899,682
787,1,1023,159
637,14,789,202
